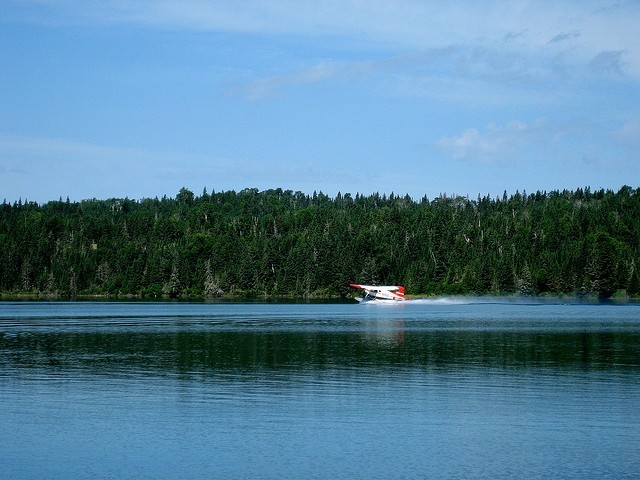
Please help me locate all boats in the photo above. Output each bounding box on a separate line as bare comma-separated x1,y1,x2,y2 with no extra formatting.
349,284,411,305
